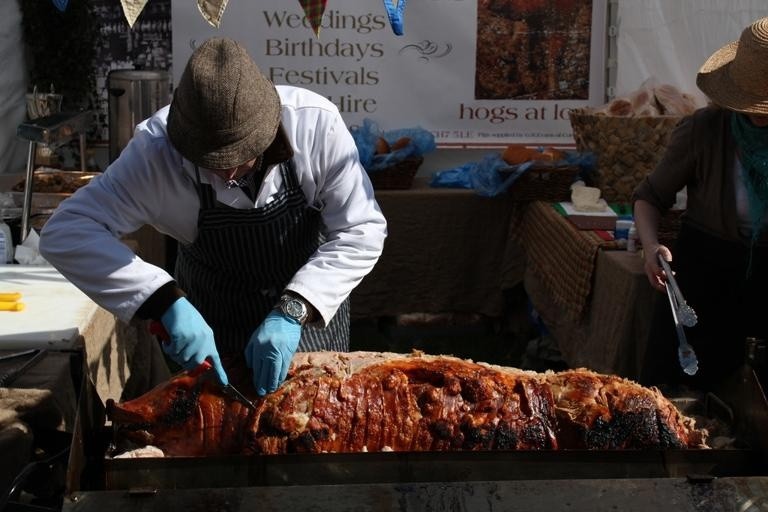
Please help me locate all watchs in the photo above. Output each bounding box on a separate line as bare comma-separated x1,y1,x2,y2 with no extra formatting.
269,293,312,329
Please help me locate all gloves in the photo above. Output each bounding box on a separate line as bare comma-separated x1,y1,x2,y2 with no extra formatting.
158,295,230,388
243,307,305,399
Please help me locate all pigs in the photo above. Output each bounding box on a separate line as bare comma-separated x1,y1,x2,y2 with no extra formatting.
104,346,693,453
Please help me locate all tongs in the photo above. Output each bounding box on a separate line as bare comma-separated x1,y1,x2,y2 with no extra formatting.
657,250,703,376
0,343,47,388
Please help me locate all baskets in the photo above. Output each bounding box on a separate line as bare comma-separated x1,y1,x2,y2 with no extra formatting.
357,151,427,191
497,162,587,203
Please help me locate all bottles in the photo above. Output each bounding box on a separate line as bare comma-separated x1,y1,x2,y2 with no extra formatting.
0,220,14,266
627,224,639,253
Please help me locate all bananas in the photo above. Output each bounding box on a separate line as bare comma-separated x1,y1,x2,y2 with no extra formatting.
0,292,22,302
0,301,25,311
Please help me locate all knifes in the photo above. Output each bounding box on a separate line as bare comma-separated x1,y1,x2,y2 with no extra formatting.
146,320,260,414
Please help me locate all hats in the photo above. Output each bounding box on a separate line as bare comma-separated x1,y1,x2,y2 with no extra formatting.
693,15,768,119
164,35,285,172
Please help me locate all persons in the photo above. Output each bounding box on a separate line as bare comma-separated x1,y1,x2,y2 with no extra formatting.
38,33,387,396
628,17,767,397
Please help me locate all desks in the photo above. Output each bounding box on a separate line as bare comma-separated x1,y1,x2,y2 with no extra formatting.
358,175,576,343
524,206,676,393
0,174,154,464
